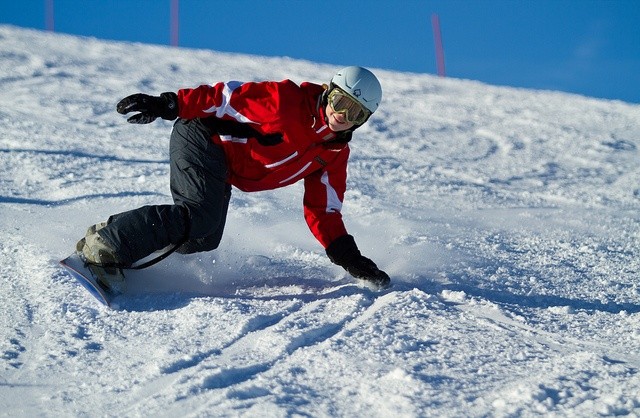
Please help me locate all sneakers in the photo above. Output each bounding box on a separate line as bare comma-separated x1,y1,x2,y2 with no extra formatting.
86,223,108,237
83,233,125,293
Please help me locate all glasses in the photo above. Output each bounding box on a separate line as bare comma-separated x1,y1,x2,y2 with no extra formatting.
327,88,370,125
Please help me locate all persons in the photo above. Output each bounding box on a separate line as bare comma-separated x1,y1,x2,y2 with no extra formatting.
73,65,394,297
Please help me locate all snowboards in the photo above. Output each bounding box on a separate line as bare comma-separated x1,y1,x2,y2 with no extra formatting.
61,249,113,307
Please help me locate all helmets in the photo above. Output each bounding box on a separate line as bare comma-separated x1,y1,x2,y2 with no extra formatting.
331,66,382,114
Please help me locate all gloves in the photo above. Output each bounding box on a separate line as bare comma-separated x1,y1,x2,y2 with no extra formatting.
324,235,390,288
116,92,179,124
